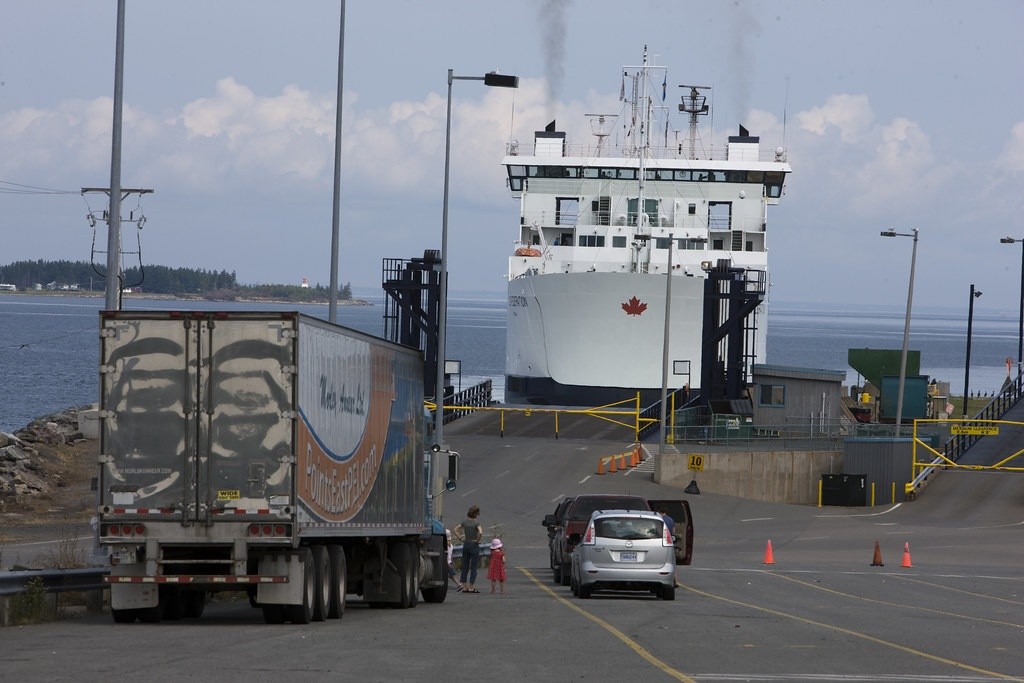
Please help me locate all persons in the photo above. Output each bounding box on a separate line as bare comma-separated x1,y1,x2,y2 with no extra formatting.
657,507,679,588
445,529,463,592
486,539,506,594
454,506,483,594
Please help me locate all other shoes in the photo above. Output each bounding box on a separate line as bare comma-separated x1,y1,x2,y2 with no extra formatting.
456,584,463,591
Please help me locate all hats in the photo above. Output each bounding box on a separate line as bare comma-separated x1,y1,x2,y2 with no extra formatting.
489,538,502,549
445,529,451,541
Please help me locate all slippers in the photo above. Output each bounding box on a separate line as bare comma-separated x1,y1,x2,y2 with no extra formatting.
463,588,480,593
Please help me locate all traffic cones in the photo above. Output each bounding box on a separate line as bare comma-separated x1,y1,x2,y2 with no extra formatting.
870,540,885,566
763,539,775,564
617,453,627,470
607,455,618,472
595,457,606,475
628,451,637,467
898,542,913,568
635,450,641,464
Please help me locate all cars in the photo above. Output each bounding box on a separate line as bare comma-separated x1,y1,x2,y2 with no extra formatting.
570,509,676,601
542,494,694,593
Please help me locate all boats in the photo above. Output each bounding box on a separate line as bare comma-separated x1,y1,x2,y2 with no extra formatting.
501,45,792,409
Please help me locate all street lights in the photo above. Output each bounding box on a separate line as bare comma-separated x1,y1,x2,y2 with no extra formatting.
963,284,983,427
432,69,519,523
880,228,918,438
1000,237,1024,396
634,233,673,454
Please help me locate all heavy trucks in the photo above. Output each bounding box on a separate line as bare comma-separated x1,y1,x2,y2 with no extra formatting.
94,310,460,625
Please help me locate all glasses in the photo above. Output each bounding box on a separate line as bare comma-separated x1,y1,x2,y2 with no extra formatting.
478,513,480,516
